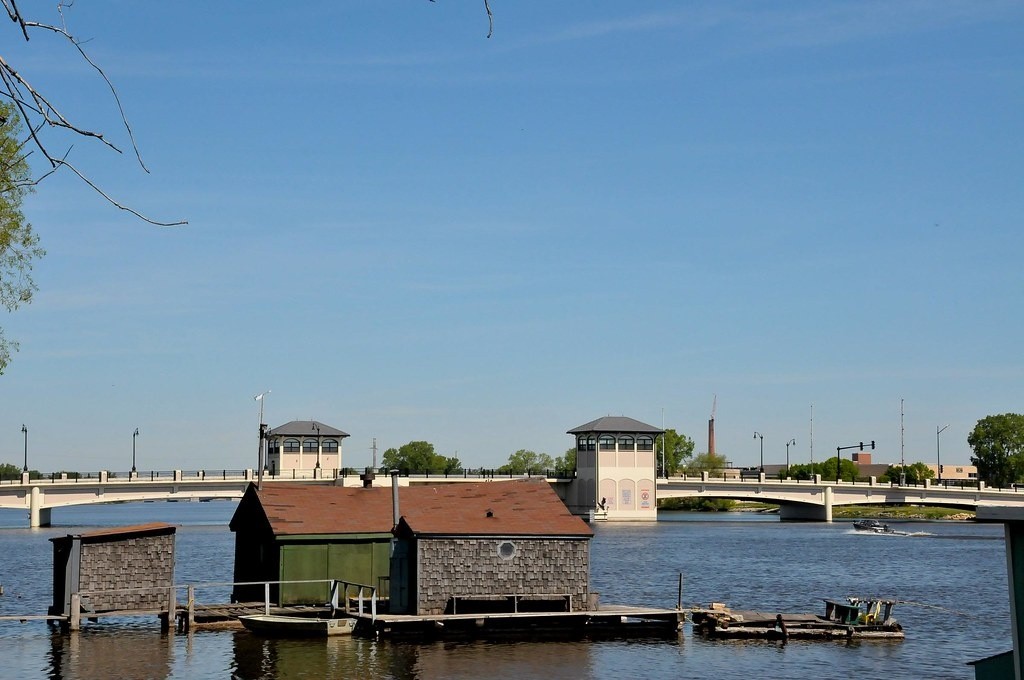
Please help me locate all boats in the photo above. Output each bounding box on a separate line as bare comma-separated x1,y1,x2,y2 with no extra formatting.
237,613,358,637
852,520,908,536
692,594,905,643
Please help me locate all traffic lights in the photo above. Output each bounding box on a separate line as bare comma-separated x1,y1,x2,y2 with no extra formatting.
859,442,863,451
872,441,875,449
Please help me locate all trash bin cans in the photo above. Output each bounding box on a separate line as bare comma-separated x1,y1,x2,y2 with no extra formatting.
899,473,905,486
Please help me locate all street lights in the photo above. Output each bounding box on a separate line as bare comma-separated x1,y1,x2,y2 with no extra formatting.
131,428,139,472
786,438,796,477
254,388,272,424
937,424,950,484
312,422,320,467
21,423,29,473
753,431,764,473
